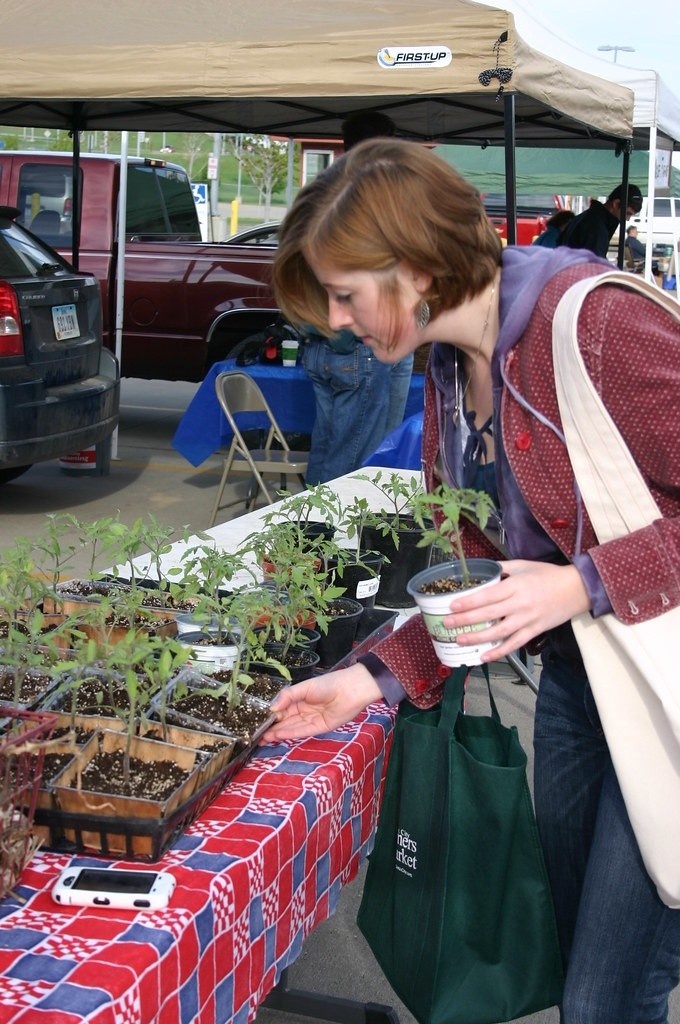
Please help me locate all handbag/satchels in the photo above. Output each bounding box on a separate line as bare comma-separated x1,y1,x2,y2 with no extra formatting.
354,661,562,1024
663,274,675,289
553,268,680,910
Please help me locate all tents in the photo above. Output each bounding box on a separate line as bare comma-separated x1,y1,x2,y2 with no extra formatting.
0,1,680,459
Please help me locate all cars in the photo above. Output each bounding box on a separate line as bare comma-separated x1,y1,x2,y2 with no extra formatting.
222,218,283,247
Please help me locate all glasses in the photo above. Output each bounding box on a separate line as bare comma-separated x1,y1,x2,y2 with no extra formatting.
627,194,641,202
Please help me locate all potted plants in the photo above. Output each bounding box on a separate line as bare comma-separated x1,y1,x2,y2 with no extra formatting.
406,482,504,668
0,470,437,900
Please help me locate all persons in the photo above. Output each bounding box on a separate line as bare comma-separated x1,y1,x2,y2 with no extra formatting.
303,110,395,491
533,211,574,249
260,138,680,1024
626,226,660,275
556,184,643,259
385,351,413,434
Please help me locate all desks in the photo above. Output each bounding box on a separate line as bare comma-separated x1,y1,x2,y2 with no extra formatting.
170,365,425,510
0,466,426,1024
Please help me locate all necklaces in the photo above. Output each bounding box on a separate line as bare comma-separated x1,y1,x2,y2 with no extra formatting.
453,280,495,427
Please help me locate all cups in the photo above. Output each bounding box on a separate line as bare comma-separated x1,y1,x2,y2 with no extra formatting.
281,339,299,367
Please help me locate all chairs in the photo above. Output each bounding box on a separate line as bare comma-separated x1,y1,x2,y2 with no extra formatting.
208,371,306,530
624,242,656,273
29,210,61,234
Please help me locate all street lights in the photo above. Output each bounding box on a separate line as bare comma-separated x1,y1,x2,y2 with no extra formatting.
599,46,635,62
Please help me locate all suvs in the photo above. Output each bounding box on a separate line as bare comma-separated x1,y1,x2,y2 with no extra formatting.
2,203,119,487
598,194,680,249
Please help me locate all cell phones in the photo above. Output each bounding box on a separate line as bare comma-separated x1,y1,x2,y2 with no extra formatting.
51,866,177,911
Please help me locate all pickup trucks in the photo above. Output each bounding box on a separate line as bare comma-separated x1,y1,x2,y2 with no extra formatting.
0,153,292,384
483,193,569,247
